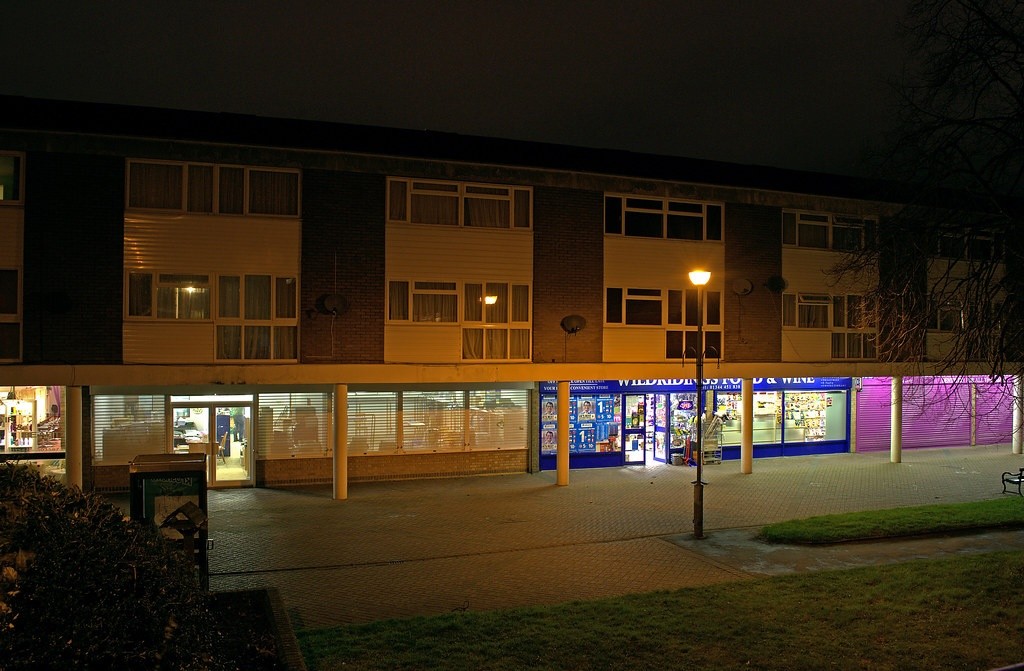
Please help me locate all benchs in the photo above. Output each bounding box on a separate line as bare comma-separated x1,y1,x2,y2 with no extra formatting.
1000,466,1024,498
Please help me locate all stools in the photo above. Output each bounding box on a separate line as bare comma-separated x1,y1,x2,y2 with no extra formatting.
353,411,368,435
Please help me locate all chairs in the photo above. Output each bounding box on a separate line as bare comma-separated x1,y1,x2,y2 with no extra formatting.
219,431,229,464
391,419,493,449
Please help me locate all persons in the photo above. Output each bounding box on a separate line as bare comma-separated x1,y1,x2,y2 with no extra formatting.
543,431,555,445
578,400,594,414
231,407,245,442
543,402,557,415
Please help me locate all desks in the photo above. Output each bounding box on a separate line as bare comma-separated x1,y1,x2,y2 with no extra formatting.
409,422,426,445
187,441,220,456
427,428,439,432
474,431,489,436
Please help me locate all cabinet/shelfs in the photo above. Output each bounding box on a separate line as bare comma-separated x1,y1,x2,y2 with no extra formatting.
700,416,724,466
644,394,657,452
716,389,827,446
0,413,33,453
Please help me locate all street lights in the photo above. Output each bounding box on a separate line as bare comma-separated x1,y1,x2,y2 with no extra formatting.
690,270,713,544
